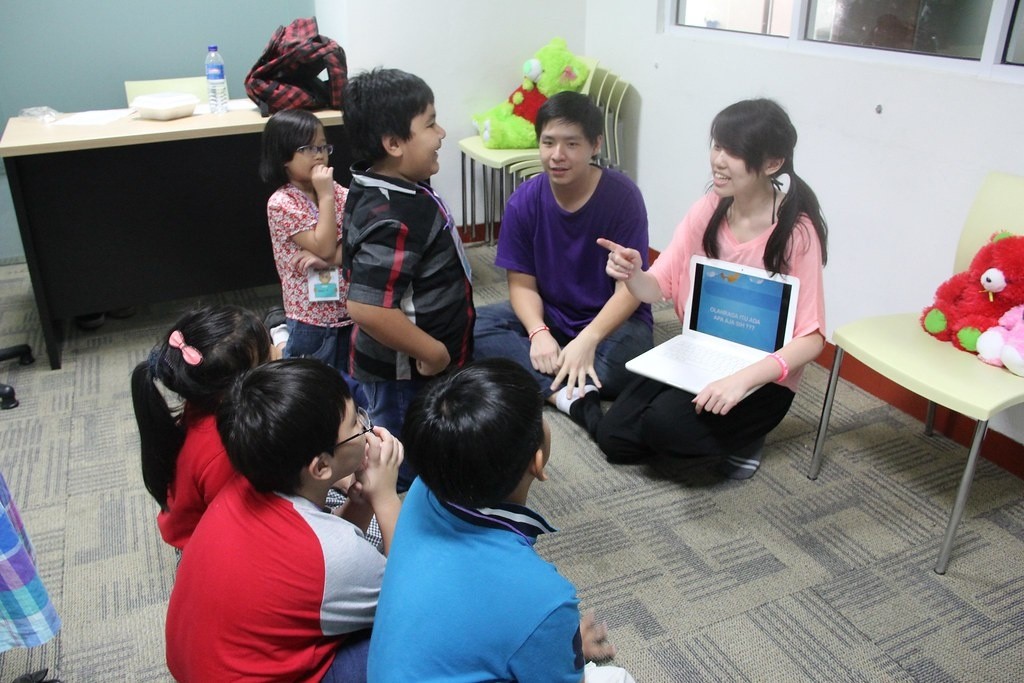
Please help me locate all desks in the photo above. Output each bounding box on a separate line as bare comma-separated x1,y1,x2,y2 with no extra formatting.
0,97,347,370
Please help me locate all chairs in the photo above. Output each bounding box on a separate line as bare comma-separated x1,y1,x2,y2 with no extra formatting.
806,170,1024,574
460,53,628,247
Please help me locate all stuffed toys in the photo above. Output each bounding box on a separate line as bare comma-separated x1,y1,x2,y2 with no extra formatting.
923,230,1024,377
475,37,592,151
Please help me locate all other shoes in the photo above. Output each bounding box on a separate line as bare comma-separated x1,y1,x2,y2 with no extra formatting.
260,305,288,343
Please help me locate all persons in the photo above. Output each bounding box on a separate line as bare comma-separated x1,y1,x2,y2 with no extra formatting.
468,92,654,440
338,69,477,493
595,99,827,482
260,110,352,372
132,302,639,683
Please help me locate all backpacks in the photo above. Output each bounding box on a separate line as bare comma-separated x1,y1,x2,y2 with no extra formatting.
243,15,349,117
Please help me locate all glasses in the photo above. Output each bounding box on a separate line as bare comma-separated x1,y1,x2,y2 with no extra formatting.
294,143,334,157
328,403,373,448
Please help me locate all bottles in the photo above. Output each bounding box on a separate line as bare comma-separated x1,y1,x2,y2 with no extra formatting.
203,44,230,118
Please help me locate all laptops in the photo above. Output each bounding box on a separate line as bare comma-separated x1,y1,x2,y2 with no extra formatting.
625,255,800,402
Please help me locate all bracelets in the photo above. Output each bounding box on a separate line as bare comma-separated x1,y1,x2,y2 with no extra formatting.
766,352,788,384
528,326,549,342
